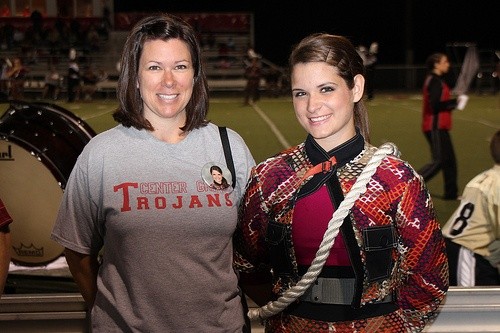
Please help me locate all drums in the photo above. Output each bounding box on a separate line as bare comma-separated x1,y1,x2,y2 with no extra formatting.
0,101,98,268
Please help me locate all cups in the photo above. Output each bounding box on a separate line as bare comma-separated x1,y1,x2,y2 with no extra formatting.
457,95,469,110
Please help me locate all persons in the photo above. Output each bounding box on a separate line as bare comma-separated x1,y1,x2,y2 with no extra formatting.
209,165,231,189
50,14,256,332
360,41,378,101
237,32,448,333
0,199,14,297
239,45,266,106
0,9,108,102
417,54,467,198
442,131,500,286
